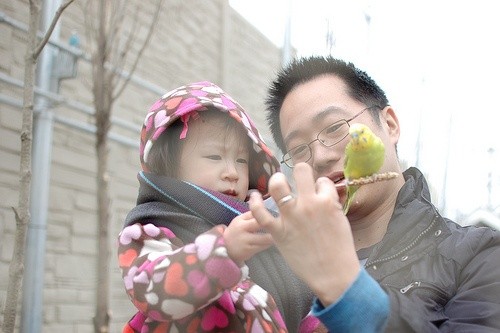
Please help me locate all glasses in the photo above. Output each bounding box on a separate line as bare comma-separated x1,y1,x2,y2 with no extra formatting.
281,103,385,169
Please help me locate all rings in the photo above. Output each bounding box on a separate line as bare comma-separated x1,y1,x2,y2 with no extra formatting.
275,194,293,206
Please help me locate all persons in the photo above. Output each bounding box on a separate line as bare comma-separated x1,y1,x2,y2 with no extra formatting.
249,53,500,333
115,79,331,333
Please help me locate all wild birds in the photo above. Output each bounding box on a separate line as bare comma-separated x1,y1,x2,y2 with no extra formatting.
342,123,386,215
